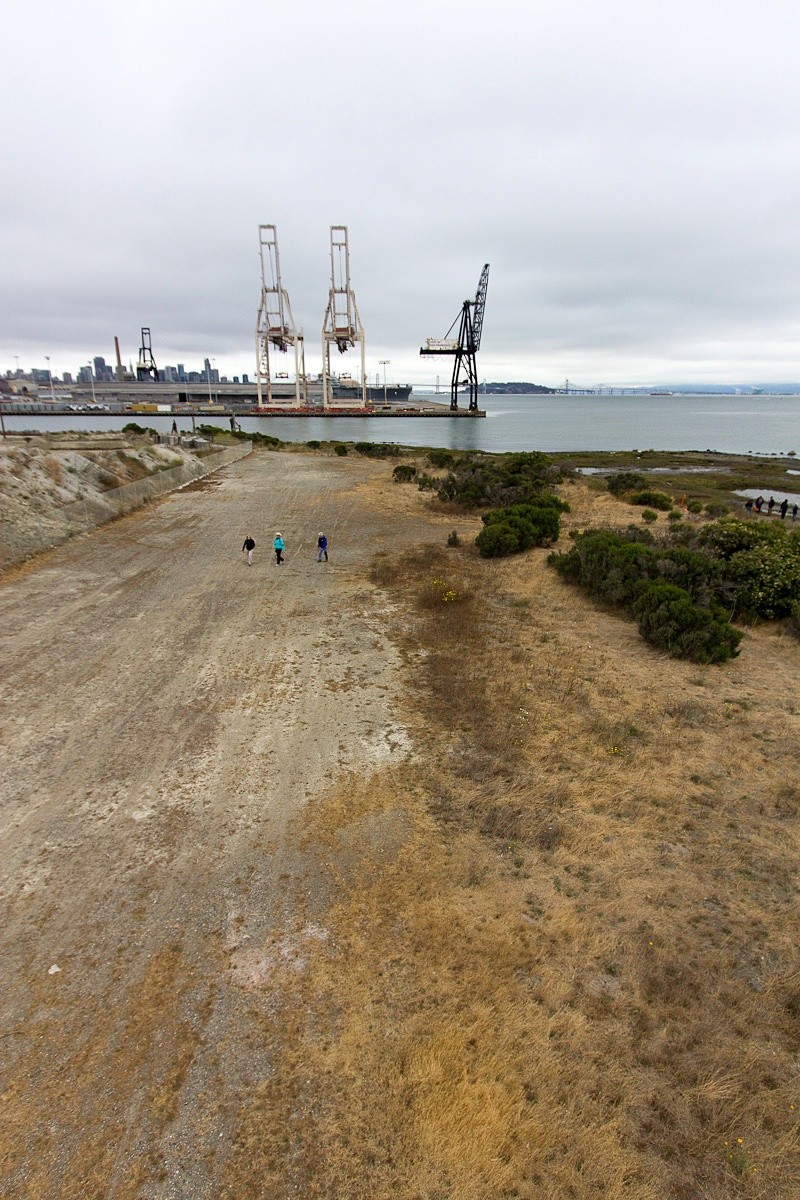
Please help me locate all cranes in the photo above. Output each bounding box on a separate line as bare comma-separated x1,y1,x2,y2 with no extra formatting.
254,223,308,409
319,225,377,411
137,327,162,382
418,263,492,411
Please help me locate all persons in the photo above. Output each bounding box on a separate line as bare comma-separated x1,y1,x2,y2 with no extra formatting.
755,495,764,514
316,532,329,562
273,532,285,567
781,499,789,519
793,503,798,520
242,536,255,566
745,499,753,515
767,496,774,516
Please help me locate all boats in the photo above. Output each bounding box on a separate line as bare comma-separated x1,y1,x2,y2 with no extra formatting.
650,392,672,395
